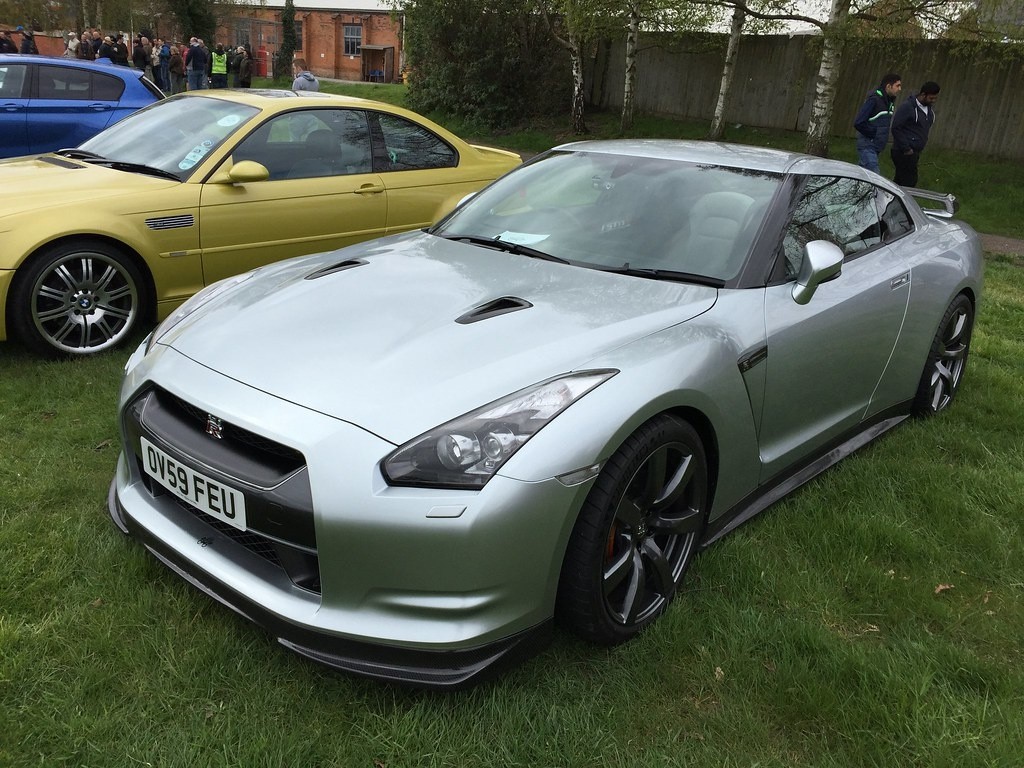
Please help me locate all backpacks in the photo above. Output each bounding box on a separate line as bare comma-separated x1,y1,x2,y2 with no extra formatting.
161,45,172,61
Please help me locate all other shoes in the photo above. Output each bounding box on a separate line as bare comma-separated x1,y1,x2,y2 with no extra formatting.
161,88,167,92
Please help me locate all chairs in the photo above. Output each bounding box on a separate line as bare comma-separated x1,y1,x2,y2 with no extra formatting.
0,67,23,98
683,191,756,277
287,129,342,175
394,133,431,169
38,72,57,98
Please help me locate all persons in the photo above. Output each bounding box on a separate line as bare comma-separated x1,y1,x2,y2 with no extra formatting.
288,58,320,114
0,29,39,54
890,81,941,188
851,74,902,174
63,31,253,95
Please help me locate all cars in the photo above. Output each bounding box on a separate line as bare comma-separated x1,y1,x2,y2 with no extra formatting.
1,67,89,98
0,51,172,161
0,85,523,357
108,135,988,686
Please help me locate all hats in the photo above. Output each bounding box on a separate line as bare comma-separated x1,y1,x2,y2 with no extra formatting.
16,26,23,31
606,178,649,207
67,31,76,36
